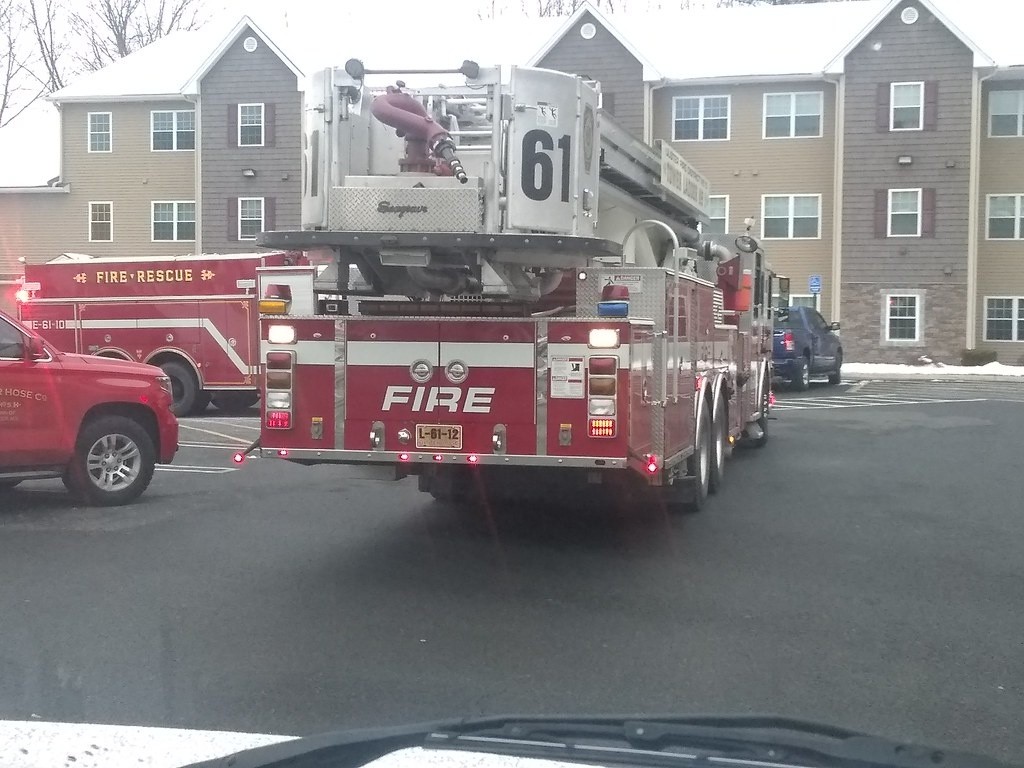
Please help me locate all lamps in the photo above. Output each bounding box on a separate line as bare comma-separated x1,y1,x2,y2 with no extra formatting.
242,168,256,177
897,156,914,163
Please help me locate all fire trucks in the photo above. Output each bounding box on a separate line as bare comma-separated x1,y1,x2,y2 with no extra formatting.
14,252,315,420
233,56,793,522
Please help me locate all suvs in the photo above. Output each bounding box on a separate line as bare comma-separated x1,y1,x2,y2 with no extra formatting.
773,305,843,391
0,308,181,508
